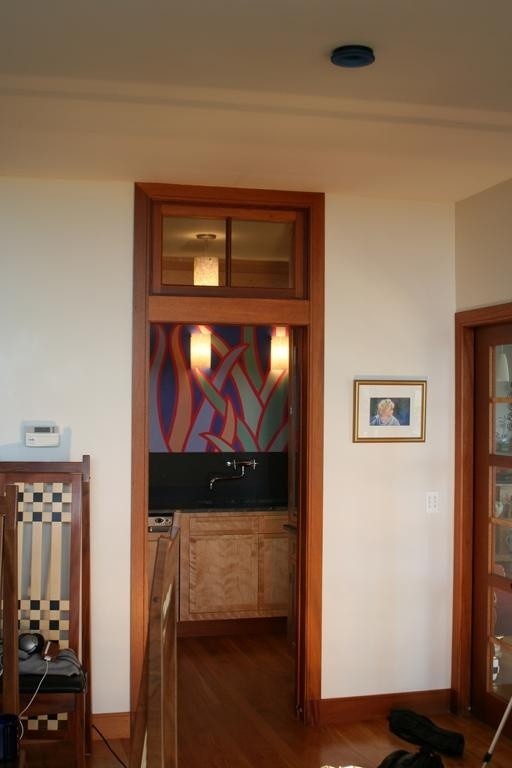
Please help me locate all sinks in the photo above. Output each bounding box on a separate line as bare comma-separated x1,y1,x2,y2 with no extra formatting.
196,502,267,508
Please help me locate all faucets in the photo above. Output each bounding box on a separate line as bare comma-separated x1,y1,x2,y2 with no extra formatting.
209,456,260,491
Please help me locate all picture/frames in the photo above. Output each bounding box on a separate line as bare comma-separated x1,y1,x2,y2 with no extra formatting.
354,381,427,443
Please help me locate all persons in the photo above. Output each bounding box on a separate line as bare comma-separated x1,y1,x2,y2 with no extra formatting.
371,398,400,426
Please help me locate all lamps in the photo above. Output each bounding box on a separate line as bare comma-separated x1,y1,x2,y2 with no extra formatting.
190,332,211,369
270,335,289,370
193,234,219,286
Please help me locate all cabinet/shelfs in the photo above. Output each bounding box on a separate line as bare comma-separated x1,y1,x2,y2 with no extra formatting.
179,517,289,623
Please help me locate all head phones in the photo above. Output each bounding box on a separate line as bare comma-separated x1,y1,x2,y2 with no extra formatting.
18,633,44,655
